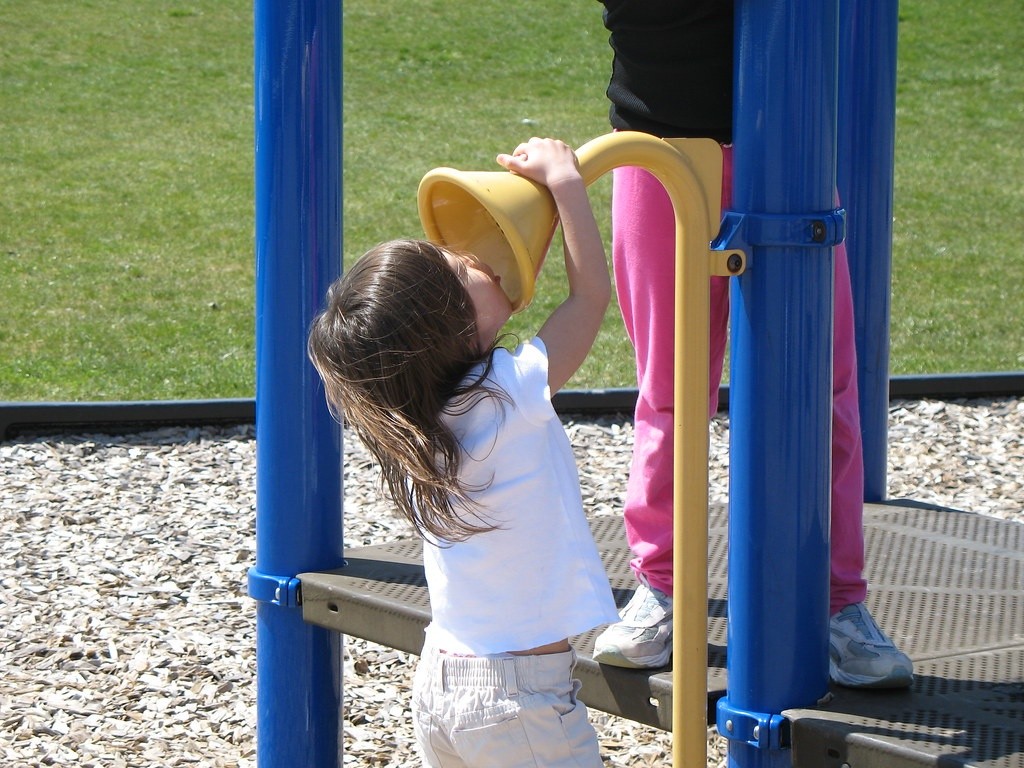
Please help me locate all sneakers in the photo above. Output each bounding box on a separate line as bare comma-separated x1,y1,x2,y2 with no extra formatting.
591,576,672,670
825,600,914,688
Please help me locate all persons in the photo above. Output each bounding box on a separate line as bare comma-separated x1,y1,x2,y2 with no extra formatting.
593,0,915,690
310,136,620,767
